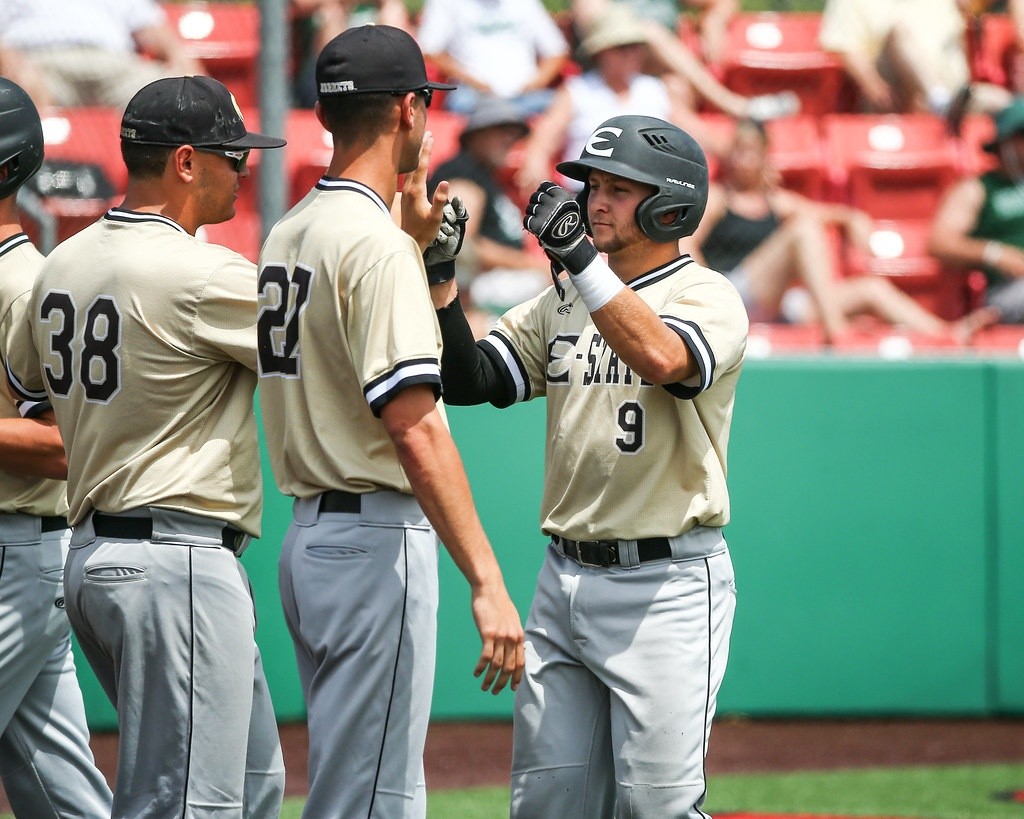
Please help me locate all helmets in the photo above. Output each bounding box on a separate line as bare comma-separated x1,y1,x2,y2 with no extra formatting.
0,77,44,200
556,115,709,244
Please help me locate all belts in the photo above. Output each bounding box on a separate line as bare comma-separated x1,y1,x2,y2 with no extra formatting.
550,533,671,567
318,489,361,514
41,516,71,533
92,513,245,558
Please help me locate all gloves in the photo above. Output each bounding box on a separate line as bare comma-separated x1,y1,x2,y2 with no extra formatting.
422,196,470,286
523,179,598,302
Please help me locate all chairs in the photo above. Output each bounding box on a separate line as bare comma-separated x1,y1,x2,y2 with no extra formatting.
18,0,1024,354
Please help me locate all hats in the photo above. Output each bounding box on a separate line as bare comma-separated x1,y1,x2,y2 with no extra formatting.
579,3,656,59
315,23,457,96
119,75,287,148
457,101,528,145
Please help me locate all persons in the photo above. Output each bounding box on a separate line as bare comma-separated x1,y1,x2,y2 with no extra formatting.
256,26,526,819
1,78,114,819
27,73,449,819
2,0,1024,355
421,114,750,819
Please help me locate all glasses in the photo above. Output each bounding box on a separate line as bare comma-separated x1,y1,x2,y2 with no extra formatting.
390,88,432,108
192,147,250,173
